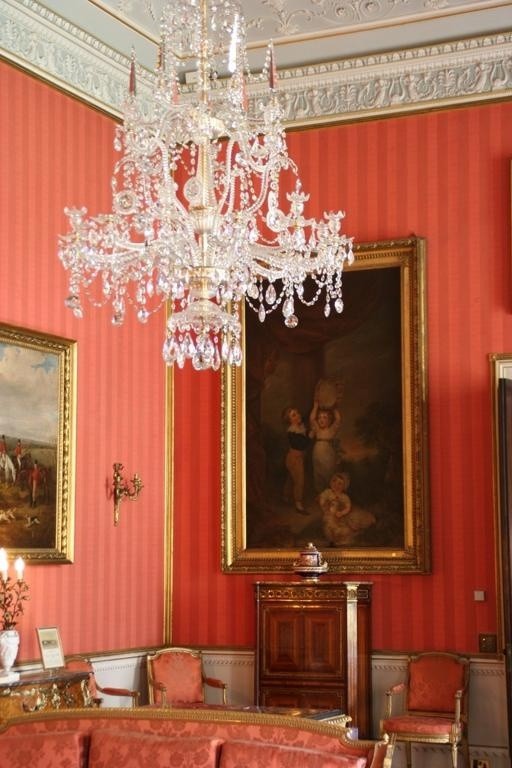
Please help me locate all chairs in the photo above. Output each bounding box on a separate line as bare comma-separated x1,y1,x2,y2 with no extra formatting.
67,654,141,709
145,647,228,706
380,650,470,767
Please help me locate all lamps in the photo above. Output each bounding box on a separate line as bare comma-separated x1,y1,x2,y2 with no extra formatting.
112,463,144,525
55,0,357,374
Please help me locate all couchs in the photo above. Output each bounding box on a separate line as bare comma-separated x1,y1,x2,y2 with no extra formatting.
0,707,392,768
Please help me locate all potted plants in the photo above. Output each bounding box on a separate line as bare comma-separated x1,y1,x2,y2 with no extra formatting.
1,575,29,672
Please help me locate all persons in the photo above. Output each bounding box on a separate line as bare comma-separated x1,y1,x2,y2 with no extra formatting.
1,431,54,512
311,395,342,498
282,405,316,515
316,470,362,547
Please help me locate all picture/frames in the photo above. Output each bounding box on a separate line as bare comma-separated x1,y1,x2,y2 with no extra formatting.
1,322,79,564
37,625,66,670
220,237,429,575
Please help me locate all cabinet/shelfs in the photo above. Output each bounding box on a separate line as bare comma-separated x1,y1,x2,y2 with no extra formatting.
253,582,371,740
